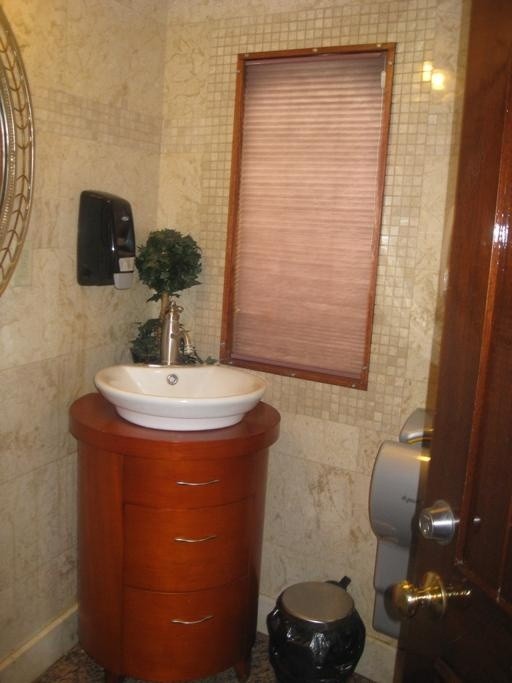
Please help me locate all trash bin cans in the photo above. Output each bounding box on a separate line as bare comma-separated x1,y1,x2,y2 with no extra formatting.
267,582,365,683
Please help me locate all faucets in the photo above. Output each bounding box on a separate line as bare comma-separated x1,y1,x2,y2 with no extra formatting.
160,301,184,365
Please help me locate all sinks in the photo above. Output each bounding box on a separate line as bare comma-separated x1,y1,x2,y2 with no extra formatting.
99,365,265,400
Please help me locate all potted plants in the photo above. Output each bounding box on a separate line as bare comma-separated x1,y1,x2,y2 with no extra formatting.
129,227,201,363
